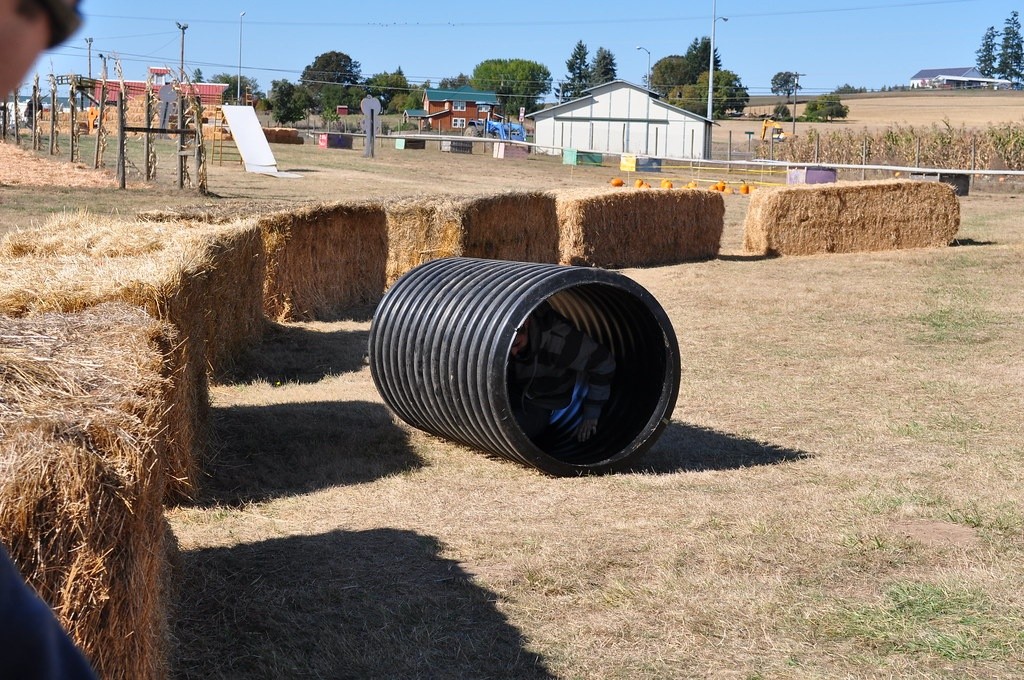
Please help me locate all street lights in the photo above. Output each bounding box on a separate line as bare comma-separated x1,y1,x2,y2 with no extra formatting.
635,46,651,90
83,36,94,109
708,0,729,120
175,21,189,190
237,10,247,104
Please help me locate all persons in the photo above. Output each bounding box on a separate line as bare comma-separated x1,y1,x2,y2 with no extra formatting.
24,93,43,133
510,310,615,443
0,1,96,680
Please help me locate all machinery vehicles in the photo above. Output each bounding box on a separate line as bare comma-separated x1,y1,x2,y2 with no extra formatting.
462,107,526,143
761,119,799,143
